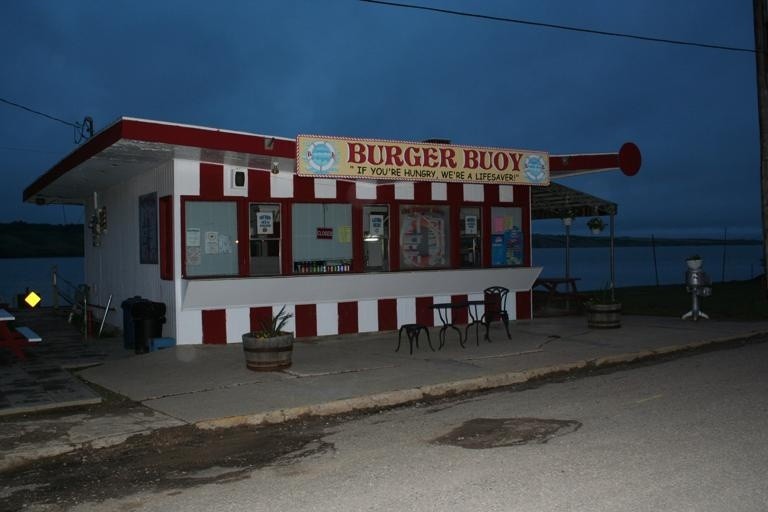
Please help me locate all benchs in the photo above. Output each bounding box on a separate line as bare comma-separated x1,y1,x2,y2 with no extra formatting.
16,326,43,347
532,292,592,303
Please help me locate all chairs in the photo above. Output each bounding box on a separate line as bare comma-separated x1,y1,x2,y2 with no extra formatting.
481,285,512,343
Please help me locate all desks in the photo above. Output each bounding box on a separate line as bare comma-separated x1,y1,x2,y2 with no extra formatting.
532,278,588,317
0,309,27,361
462,298,492,347
430,302,466,350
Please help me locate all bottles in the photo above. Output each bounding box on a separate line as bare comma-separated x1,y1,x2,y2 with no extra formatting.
296,261,350,271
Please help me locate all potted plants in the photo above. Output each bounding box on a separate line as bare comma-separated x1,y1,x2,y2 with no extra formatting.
685,254,705,270
586,215,607,234
581,280,623,329
560,209,577,226
240,303,297,371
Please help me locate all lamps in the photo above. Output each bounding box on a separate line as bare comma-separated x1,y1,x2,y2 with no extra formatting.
272,161,280,174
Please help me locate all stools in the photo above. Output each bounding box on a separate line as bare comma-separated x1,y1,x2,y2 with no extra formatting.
396,324,436,354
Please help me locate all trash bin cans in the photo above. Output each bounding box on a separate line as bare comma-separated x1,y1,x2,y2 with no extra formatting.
121,296,166,355
